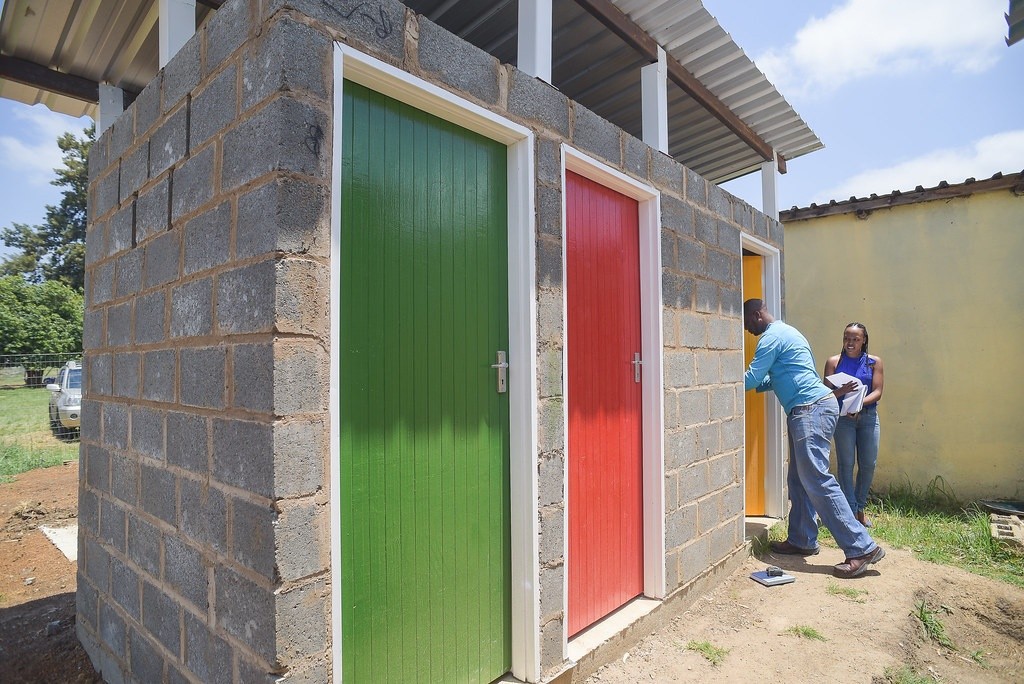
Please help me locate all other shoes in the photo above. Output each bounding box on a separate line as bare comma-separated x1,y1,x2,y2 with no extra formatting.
771,541,820,556
860,519,872,527
833,547,885,577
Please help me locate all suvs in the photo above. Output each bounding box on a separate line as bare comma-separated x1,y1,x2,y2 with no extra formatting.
46,361,82,436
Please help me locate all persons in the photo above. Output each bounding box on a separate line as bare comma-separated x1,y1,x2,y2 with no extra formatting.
744,298,886,580
824,322,884,527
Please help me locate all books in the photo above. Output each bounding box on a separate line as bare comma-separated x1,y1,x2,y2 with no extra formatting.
749,569,795,587
